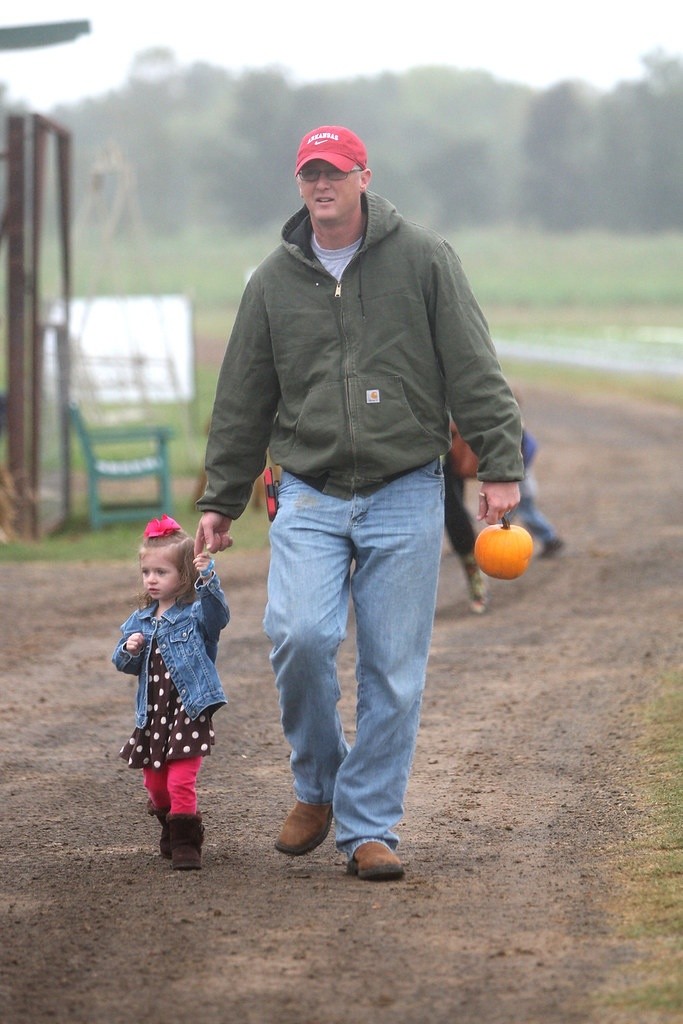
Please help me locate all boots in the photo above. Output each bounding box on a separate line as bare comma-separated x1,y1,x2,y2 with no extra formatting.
147,798,173,859
166,812,203,869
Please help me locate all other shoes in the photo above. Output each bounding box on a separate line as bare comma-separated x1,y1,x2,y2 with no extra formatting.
347,841,404,878
274,801,334,855
537,537,565,558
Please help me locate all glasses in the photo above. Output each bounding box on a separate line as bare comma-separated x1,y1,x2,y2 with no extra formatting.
299,165,363,181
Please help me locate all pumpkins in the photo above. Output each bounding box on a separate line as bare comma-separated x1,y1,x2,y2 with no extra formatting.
474,524,533,579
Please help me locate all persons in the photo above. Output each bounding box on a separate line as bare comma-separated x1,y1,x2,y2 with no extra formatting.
444,408,564,617
195,126,527,878
111,515,230,869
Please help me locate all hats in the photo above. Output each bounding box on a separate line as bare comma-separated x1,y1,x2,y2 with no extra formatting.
294,125,369,176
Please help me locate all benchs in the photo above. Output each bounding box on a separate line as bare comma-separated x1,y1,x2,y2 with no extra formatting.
67,399,175,534
